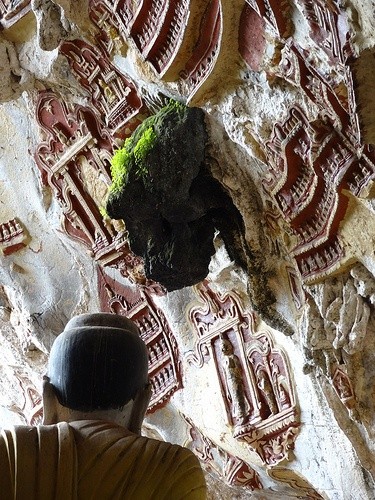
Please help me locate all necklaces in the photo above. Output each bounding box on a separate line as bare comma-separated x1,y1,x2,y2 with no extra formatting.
105,0,216,96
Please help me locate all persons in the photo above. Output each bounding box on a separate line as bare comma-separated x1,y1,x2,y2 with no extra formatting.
337,377,349,399
96,78,115,105
217,332,251,426
0,218,22,241
1,313,207,500
255,110,375,283
132,318,178,403
257,370,277,418
